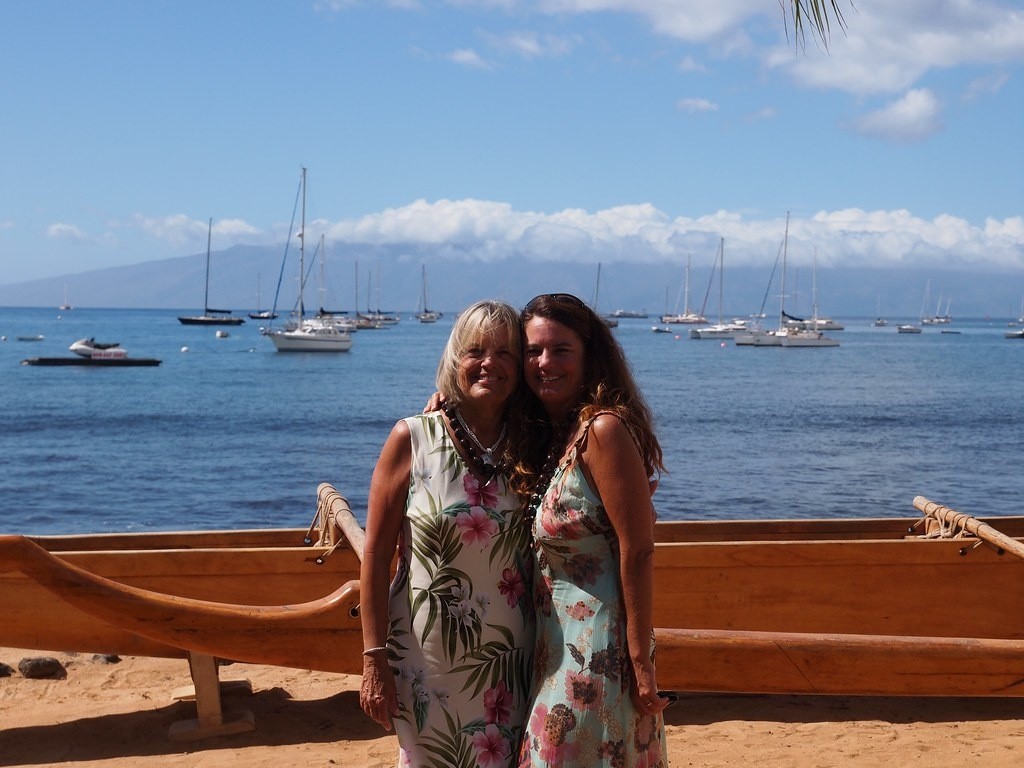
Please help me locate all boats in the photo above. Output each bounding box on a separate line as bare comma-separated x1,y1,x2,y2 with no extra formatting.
20,337,163,367
652,326,673,333
612,307,649,319
1004,327,1024,339
17,335,45,342
2,491,1021,703
897,325,924,334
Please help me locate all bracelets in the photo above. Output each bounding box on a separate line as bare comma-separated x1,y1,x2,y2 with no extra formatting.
363,646,389,654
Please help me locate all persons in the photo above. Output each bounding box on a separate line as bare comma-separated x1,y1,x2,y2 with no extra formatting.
359,298,539,768
514,293,672,768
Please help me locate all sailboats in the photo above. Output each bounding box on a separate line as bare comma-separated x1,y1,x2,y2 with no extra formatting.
248,271,279,319
261,167,351,353
284,234,358,333
781,247,845,332
734,207,836,348
874,293,888,326
915,278,953,325
587,262,619,327
657,252,710,325
176,216,246,326
356,261,444,330
690,235,751,339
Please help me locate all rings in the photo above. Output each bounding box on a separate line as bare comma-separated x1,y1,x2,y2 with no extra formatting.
646,701,652,707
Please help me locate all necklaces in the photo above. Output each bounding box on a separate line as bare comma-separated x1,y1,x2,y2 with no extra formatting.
520,416,570,525
440,399,519,474
